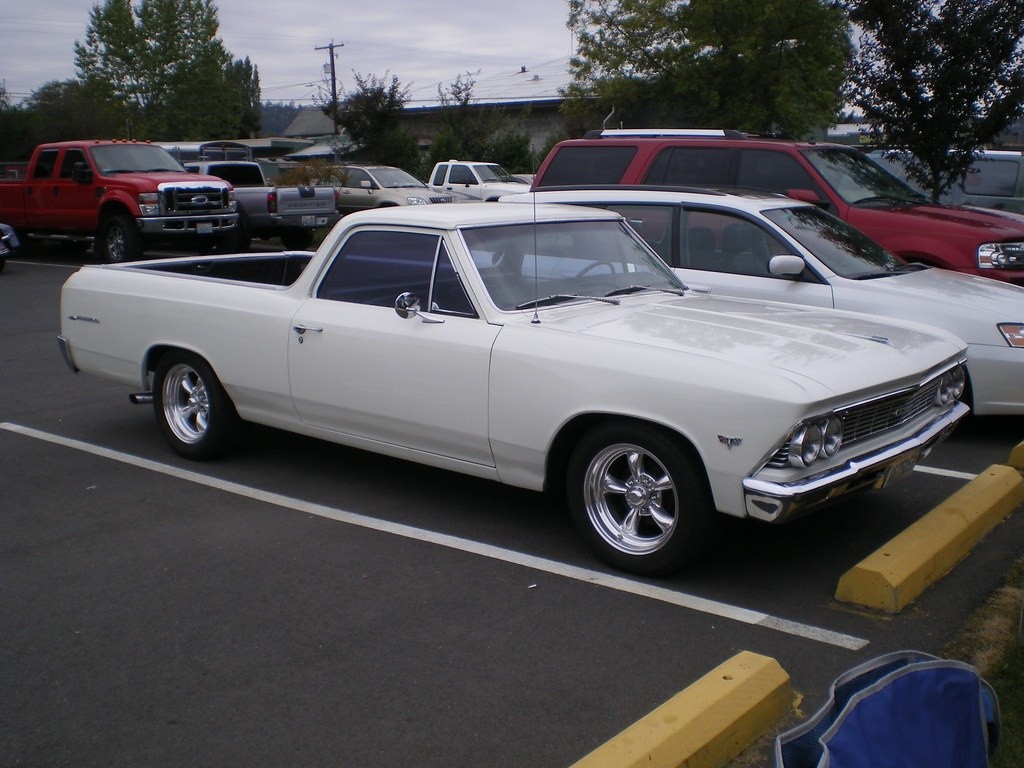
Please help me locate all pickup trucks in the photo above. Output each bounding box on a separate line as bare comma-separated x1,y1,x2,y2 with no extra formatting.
0,137,239,265
56,200,972,576
395,159,531,202
525,127,1024,289
180,162,335,251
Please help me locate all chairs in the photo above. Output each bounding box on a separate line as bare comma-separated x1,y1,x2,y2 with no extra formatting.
688,227,721,264
723,223,771,260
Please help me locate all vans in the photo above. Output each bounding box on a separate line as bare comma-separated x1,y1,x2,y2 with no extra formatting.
308,165,457,218
863,147,1024,214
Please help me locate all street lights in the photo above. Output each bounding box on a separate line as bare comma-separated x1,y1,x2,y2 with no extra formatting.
305,83,338,137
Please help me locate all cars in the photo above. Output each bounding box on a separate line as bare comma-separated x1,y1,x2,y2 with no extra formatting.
501,184,1024,432
